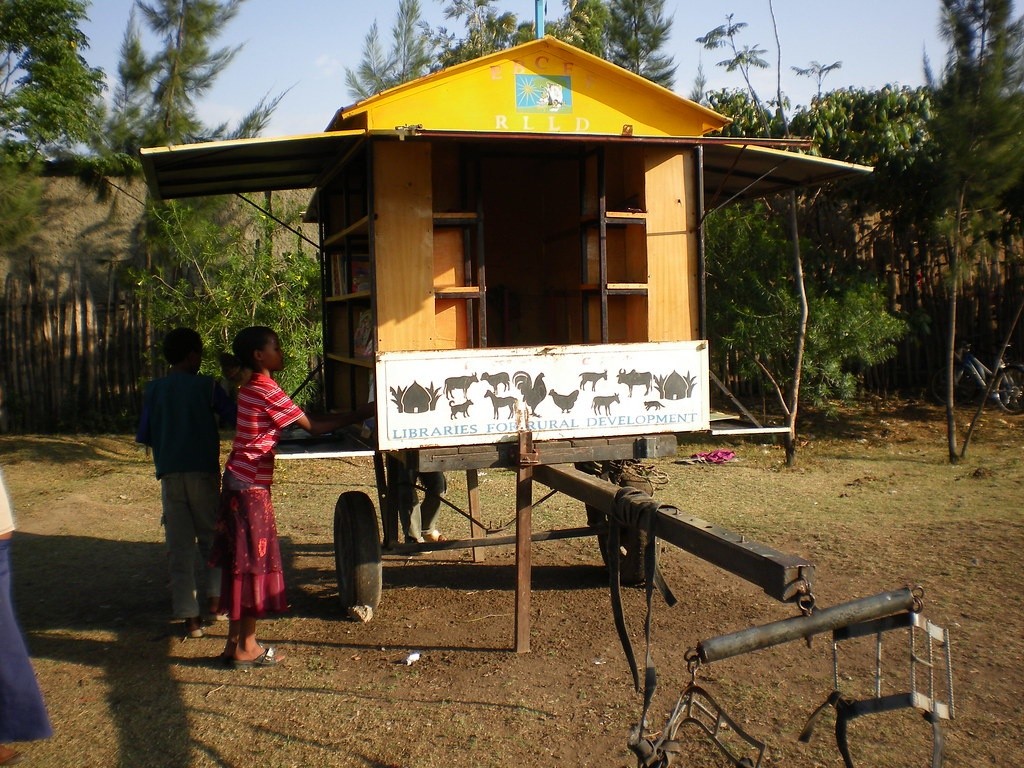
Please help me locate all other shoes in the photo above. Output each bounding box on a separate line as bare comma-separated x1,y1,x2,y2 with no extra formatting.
424,529,448,542
0,744,25,767
203,607,229,625
404,537,432,555
184,618,203,637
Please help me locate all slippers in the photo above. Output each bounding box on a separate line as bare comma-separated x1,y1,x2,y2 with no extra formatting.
221,640,290,668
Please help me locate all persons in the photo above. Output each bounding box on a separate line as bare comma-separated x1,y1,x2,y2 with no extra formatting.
135,328,237,638
0,475,52,764
207,326,375,669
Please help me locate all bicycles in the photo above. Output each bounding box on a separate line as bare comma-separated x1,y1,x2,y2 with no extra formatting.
928,342,1024,416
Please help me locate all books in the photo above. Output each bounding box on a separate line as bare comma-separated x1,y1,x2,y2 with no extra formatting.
331,253,370,298
